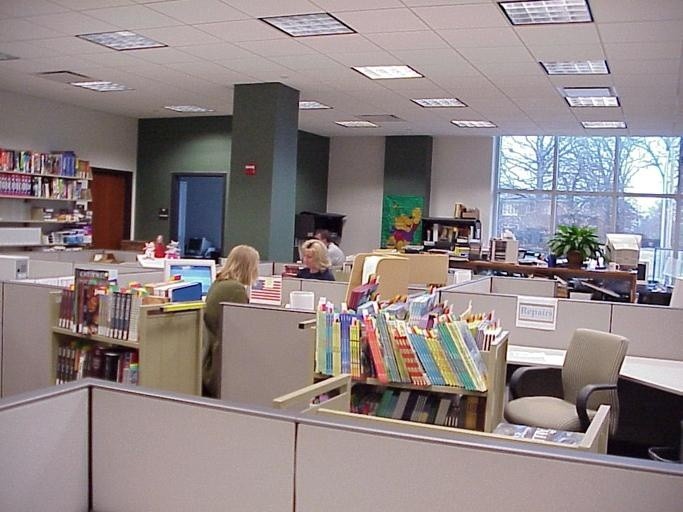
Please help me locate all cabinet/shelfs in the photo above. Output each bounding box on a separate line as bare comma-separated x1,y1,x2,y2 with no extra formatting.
415,211,483,261
293,207,345,264
0,148,95,249
312,311,510,436
44,286,169,390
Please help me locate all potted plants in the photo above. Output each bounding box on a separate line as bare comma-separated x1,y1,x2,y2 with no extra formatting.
548,223,606,269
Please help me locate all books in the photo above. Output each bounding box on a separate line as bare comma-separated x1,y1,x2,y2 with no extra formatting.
313,273,503,432
427,204,474,242
55,267,203,385
0,147,93,246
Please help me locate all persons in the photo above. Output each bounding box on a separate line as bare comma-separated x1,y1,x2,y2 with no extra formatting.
295,239,335,281
203,245,260,337
312,229,345,265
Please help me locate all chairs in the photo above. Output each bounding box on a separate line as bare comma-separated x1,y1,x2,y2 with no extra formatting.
503,326,630,442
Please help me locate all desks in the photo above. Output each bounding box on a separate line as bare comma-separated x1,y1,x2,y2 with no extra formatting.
509,342,683,396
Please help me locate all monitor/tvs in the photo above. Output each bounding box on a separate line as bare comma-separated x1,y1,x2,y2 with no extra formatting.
186,235,214,255
636,260,649,285
604,233,642,269
164,259,217,304
